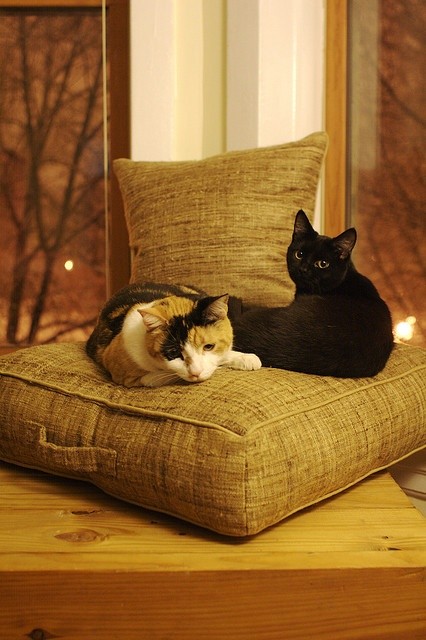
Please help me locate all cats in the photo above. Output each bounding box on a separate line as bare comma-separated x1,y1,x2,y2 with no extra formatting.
85,279,262,389
222,209,395,379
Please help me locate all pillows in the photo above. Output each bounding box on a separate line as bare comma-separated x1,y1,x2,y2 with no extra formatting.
112,130,330,309
1,333,426,537
